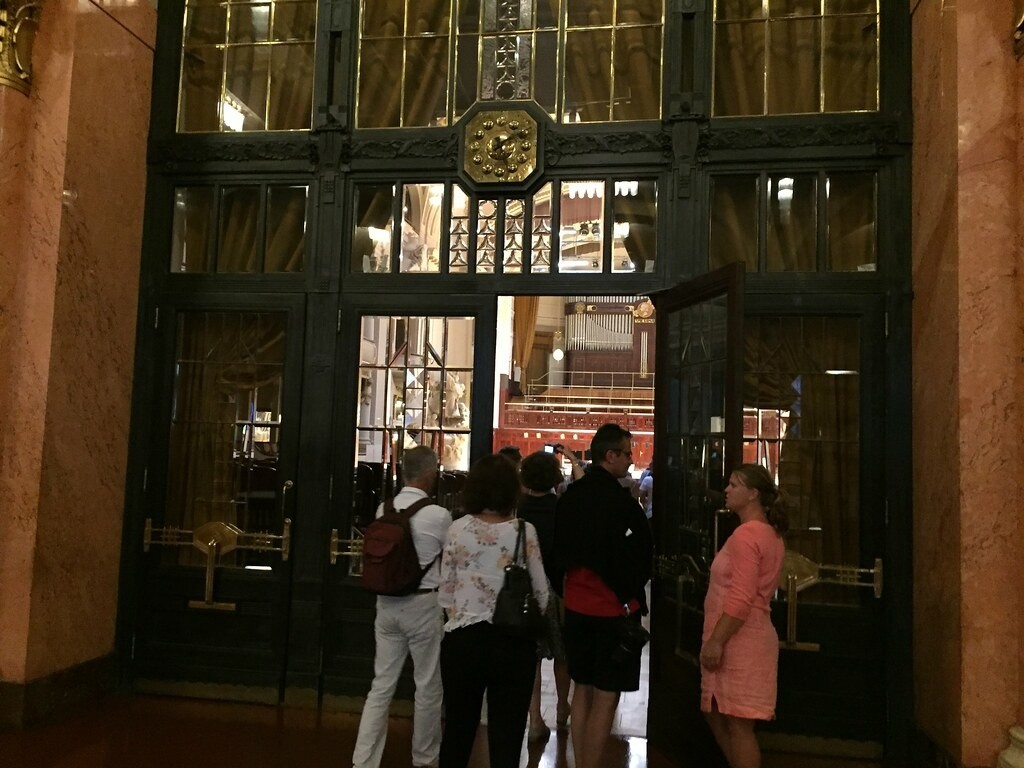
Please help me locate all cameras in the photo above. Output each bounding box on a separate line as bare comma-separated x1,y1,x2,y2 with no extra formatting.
545,445,559,454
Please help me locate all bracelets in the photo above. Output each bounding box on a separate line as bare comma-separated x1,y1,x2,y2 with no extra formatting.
572,463,579,466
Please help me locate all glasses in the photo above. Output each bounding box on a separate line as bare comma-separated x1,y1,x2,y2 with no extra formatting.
555,450,564,455
608,447,631,460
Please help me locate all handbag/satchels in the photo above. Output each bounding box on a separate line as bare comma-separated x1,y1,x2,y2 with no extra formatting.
493,519,541,641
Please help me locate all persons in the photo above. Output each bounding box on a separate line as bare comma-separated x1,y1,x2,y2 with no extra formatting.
437,422,654,768
700,463,790,768
352,446,453,768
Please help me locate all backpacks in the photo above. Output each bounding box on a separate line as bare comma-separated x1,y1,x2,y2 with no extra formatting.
365,499,442,596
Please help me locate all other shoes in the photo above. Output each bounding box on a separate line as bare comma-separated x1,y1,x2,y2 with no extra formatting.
557,701,570,725
528,724,550,743
